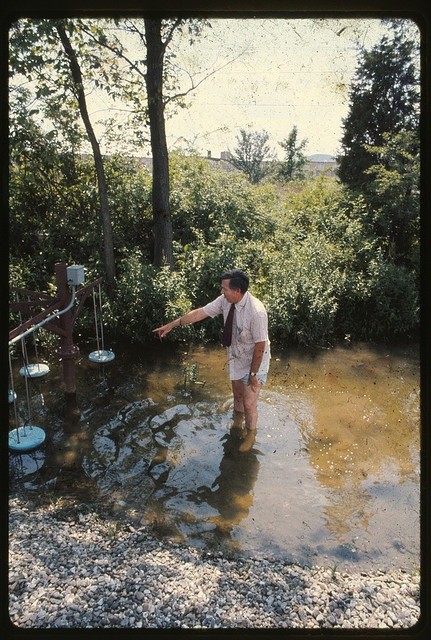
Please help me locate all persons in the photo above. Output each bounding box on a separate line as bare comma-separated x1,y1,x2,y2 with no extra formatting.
153,269,271,454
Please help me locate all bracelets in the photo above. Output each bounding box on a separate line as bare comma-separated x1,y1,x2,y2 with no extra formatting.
179,316,183,328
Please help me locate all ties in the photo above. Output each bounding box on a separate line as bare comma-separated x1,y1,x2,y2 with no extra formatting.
222,304,234,346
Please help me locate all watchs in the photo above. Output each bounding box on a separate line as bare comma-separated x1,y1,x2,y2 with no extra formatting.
249,372,257,377
